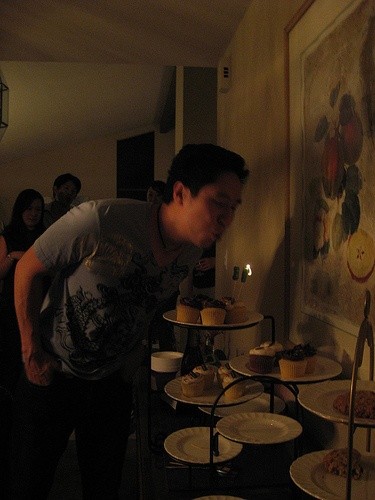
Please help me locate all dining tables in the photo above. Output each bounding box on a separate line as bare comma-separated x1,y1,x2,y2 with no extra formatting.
133,362,321,500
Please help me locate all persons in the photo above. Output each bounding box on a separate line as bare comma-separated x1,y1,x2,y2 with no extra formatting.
43,173,81,233
5,144,250,499
0,189,46,404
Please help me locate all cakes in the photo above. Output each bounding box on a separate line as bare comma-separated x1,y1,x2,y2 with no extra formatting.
247,341,317,378
176,293,228,325
181,363,245,400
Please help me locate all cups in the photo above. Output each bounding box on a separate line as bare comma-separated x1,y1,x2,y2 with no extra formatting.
151,351,183,391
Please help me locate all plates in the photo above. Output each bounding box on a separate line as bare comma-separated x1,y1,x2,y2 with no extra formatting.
164,352,375,500
162,306,266,327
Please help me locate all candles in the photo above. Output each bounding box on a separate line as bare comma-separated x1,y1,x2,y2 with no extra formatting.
232,265,240,281
241,267,249,282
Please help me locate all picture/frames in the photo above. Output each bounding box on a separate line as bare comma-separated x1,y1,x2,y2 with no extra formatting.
280,0,375,387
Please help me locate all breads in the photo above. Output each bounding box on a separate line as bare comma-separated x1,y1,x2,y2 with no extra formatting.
323,447,363,480
332,390,375,419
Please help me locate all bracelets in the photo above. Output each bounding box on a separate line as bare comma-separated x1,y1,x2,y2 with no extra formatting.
6,252,13,262
